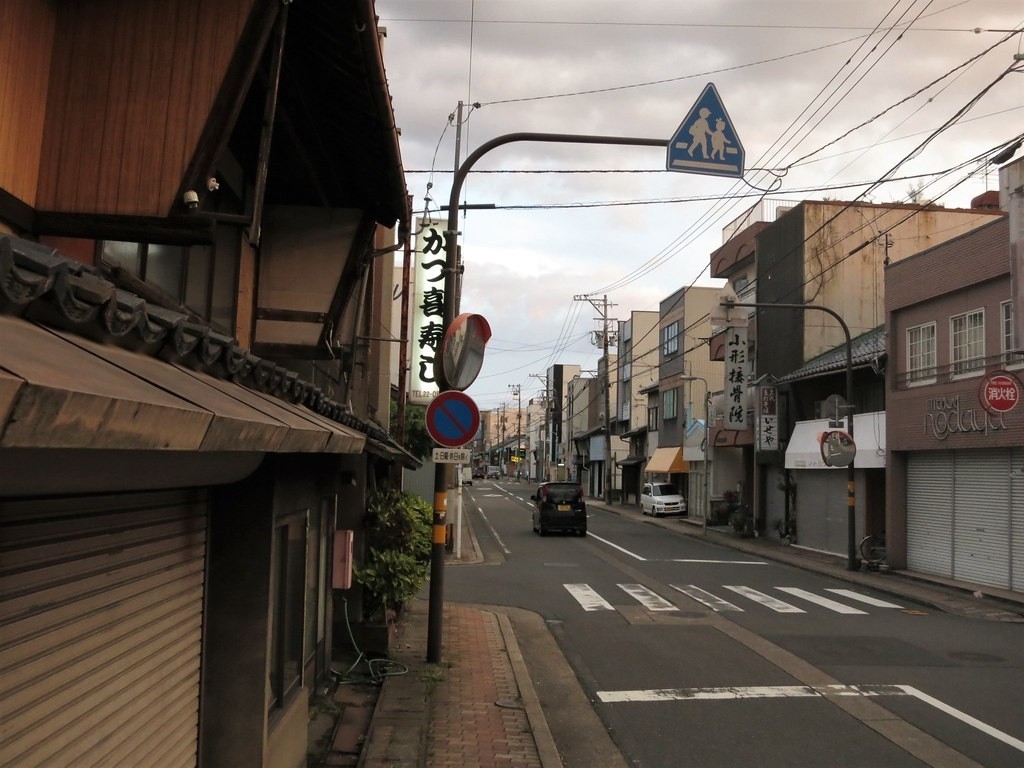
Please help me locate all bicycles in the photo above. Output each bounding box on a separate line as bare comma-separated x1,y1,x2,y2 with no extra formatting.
860,531,886,562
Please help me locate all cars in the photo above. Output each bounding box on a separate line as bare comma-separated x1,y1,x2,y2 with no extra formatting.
640,481,687,518
472,467,485,480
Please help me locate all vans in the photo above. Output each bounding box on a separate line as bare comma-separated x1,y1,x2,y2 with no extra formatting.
531,480,588,538
486,465,500,480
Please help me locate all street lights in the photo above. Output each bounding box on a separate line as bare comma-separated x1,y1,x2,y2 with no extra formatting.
680,374,711,534
